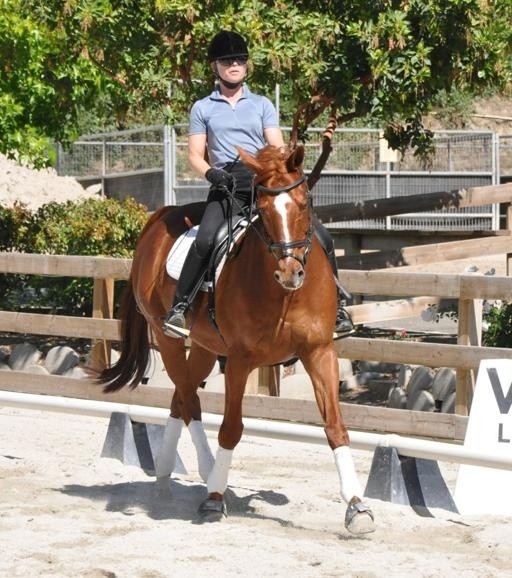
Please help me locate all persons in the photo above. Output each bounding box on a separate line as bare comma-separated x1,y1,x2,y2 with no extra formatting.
163,29,354,341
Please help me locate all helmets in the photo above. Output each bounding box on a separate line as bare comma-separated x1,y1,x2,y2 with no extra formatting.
208,30,249,63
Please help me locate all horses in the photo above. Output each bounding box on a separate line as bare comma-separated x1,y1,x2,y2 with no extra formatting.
76,144,376,535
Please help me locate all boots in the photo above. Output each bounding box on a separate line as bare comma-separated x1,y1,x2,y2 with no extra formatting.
324,248,354,333
163,240,214,339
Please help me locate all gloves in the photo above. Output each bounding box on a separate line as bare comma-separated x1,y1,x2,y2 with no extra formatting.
204,168,233,191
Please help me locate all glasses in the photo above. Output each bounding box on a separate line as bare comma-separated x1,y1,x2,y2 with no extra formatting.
217,56,248,66
